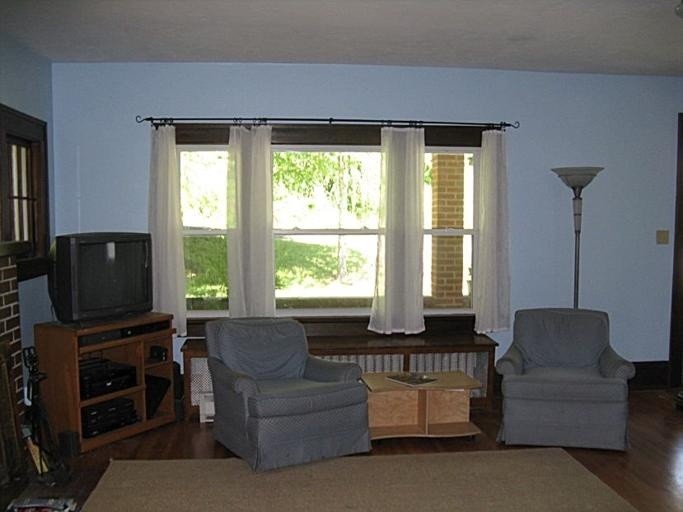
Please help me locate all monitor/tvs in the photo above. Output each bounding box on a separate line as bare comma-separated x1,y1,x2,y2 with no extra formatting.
48,232,153,321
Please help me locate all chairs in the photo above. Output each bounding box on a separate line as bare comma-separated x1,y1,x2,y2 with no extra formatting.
205,312,374,473
493,306,636,453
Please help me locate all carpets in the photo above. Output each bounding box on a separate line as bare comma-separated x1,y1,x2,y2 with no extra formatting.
75,441,621,512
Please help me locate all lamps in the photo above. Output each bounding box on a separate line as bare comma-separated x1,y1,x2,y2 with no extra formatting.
549,165,605,308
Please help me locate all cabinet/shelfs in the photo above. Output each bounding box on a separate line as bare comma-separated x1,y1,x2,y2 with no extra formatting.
32,311,177,466
